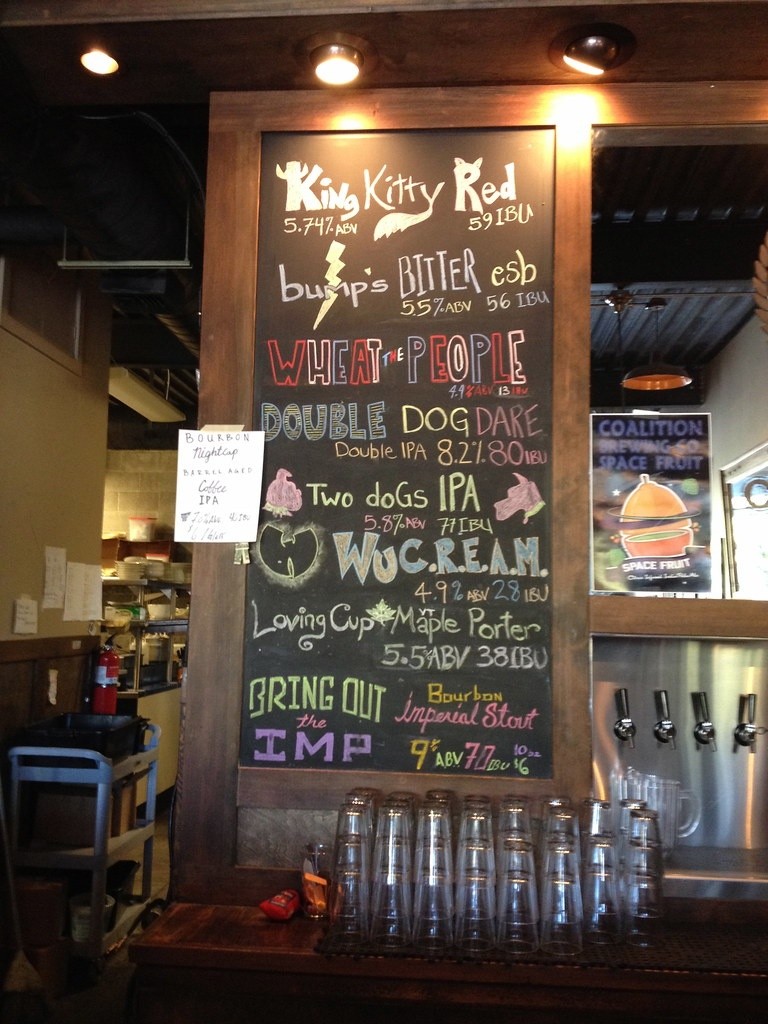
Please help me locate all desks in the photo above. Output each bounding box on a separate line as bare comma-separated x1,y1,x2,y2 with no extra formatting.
128,896,768,1024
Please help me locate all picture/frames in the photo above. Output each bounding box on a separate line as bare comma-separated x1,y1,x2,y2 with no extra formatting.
166,80,768,902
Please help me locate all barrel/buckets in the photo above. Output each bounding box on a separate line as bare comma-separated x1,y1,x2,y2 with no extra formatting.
145,638,168,662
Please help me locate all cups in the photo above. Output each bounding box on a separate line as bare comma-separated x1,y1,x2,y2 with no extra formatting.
300,787,662,956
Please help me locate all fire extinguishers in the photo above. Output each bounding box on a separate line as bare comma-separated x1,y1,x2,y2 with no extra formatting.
92,644,120,716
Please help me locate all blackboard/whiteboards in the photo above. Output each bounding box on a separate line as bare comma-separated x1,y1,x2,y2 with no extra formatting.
203,84,598,815
588,412,724,600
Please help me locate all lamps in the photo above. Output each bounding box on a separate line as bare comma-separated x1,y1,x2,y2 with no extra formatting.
314,44,363,85
620,297,692,390
563,34,624,76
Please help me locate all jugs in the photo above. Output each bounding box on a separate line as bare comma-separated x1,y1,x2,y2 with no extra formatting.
608,767,702,868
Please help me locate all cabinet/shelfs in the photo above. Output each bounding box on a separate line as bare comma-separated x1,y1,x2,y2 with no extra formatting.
8,723,163,983
102,578,192,806
0,635,101,727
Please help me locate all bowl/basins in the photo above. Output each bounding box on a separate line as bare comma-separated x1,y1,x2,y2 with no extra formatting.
149,604,171,620
115,558,191,582
